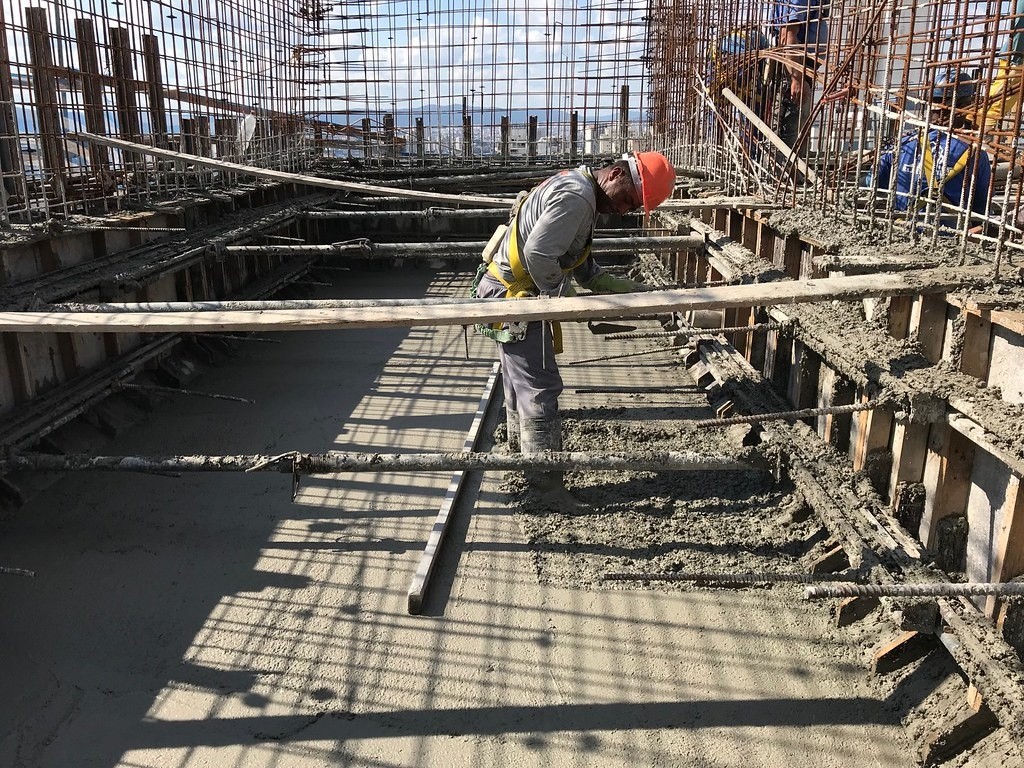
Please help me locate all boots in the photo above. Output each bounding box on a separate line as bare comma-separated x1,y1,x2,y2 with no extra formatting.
506,410,589,514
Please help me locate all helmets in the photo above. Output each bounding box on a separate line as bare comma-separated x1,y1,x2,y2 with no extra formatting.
634,149,677,222
931,68,974,99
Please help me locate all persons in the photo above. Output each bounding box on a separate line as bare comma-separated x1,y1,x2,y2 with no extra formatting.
866,67,996,234
769,0,831,188
705,21,767,177
952,0,1023,139
474,146,677,513
